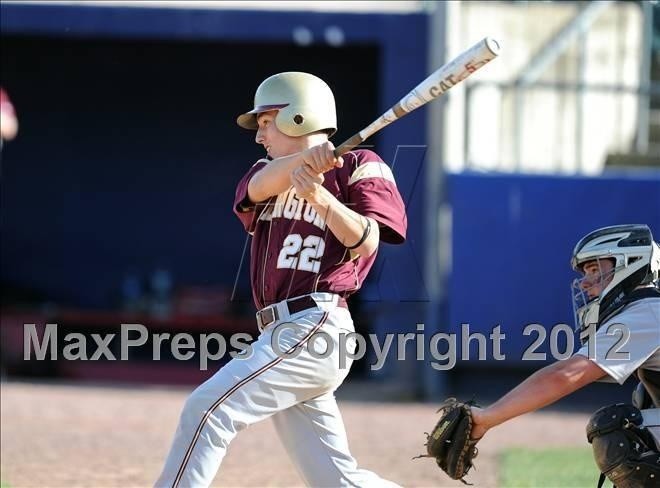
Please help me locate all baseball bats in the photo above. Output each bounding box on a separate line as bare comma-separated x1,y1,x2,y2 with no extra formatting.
333,35,500,157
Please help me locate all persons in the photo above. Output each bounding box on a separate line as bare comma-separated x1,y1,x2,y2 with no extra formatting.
152,72,407,488
426,223,660,488
1,86,20,140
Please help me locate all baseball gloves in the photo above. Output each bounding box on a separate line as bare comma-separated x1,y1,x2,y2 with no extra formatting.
427,396,480,480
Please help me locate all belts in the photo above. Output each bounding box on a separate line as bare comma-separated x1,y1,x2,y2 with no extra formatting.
257,295,318,330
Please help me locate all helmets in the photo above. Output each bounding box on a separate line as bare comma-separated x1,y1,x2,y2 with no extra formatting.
236,71,338,139
570,223,660,333
